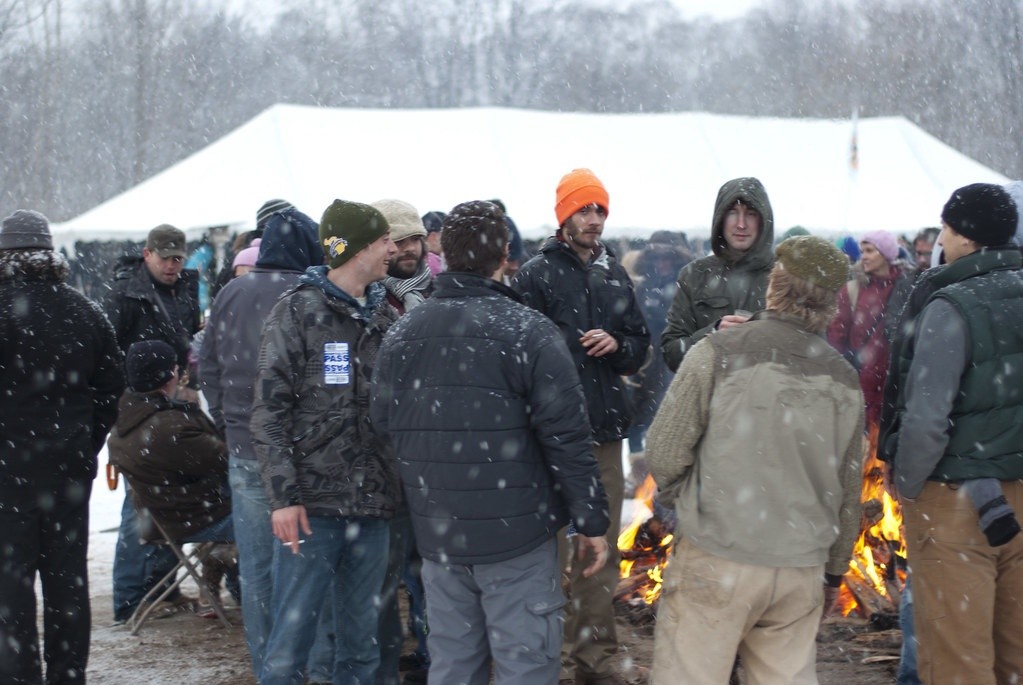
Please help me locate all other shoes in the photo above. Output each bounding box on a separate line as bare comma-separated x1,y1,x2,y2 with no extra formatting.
400,651,430,685
575,663,651,685
125,591,198,621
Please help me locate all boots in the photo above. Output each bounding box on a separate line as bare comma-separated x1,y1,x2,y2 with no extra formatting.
194,543,237,618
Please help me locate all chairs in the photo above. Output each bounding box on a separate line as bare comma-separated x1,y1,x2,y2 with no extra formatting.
112,460,234,638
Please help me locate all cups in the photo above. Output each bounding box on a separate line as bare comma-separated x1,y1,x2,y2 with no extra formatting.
735,309,755,321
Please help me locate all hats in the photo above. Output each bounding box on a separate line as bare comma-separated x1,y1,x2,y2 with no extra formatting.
319,198,390,269
860,230,899,262
256,199,298,232
255,211,323,269
773,235,848,292
424,212,447,231
555,167,610,225
942,182,1019,252
1004,183,1023,246
372,200,427,243
0,209,54,252
125,341,177,393
146,224,188,260
230,238,262,270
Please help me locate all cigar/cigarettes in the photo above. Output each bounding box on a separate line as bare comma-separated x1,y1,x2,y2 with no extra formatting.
587,333,605,339
282,540,306,546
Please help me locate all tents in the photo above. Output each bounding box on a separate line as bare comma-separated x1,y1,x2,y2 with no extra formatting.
50,75,1023,275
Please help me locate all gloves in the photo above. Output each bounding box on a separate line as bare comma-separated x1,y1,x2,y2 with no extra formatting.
965,476,1021,547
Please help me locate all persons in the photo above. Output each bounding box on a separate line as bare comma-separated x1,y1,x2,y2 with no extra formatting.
0,169,1023,684
